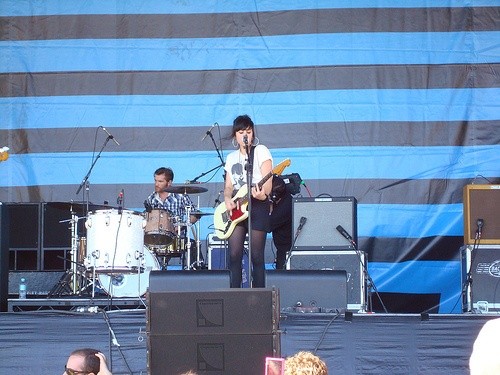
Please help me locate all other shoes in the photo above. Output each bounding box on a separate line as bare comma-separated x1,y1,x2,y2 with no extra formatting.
184,261,208,270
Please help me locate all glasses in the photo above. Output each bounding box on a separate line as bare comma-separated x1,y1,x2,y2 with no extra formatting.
64,364,91,375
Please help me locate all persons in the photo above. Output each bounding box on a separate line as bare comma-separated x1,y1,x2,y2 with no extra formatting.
224,116,273,288
144,168,206,270
269,176,293,269
62,349,113,375
283,351,328,375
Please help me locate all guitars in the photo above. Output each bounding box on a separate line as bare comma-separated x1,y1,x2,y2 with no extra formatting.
213,157,291,240
0,146,10,162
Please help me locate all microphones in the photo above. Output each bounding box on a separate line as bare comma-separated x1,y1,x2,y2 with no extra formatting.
101,126,121,147
477,218,484,238
296,217,307,233
200,125,215,143
336,225,355,245
242,136,251,142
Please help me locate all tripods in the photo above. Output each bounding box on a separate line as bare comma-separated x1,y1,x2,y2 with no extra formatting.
46,138,113,300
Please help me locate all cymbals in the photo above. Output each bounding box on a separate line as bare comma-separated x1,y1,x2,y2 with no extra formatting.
163,185,209,195
88,204,128,211
182,210,214,216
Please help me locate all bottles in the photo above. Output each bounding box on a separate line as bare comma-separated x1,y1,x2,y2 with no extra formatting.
18,278,26,299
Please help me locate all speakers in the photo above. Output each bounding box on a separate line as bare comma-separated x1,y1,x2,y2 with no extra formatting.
148,270,231,293
459,184,500,245
4,201,95,271
264,269,348,319
291,196,358,249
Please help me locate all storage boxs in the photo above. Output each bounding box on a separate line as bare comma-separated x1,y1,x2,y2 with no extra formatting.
460,245,500,314
209,245,251,288
285,250,368,311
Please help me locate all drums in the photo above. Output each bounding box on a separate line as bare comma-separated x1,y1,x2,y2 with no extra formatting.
95,245,160,298
156,234,183,257
83,208,146,275
142,208,175,248
